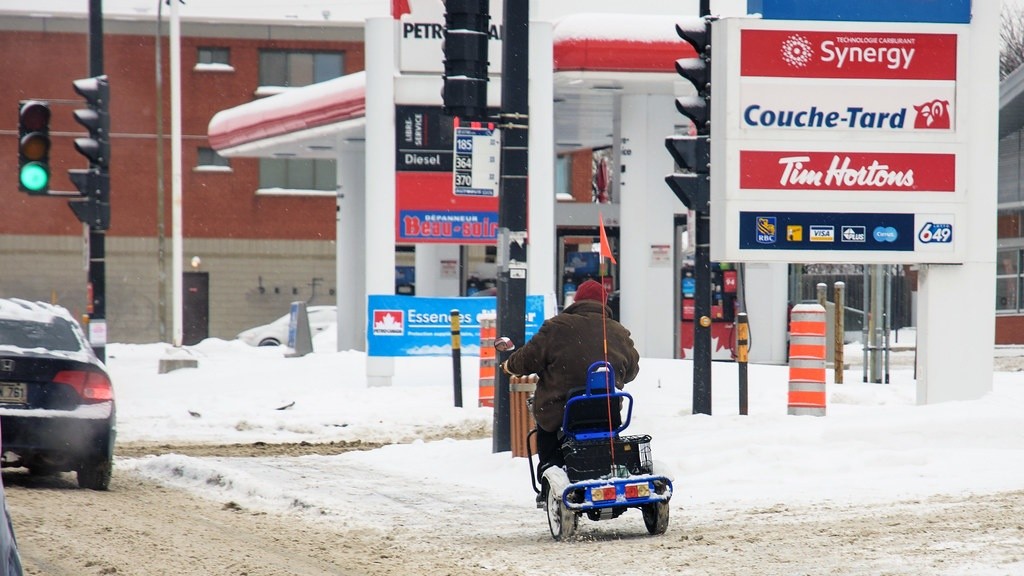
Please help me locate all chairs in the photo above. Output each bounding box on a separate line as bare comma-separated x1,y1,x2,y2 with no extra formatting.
558,362,634,443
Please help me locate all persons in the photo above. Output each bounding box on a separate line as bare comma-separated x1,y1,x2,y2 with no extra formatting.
503,280,640,464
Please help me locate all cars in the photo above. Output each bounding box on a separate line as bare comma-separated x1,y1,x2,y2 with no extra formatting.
0,297,117,492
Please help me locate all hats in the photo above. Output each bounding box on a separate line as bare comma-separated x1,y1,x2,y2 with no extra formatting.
495,338,506,346
573,280,607,305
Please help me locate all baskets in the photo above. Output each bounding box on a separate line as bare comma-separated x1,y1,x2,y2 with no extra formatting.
562,434,653,479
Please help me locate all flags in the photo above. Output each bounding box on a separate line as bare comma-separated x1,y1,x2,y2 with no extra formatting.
599,214,617,264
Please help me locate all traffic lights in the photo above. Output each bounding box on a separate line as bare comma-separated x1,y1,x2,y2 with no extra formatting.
16,99,52,196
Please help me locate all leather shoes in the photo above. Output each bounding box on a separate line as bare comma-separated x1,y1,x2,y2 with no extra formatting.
536,493,548,502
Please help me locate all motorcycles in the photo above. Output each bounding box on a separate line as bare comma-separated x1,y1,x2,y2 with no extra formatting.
491,335,677,540
